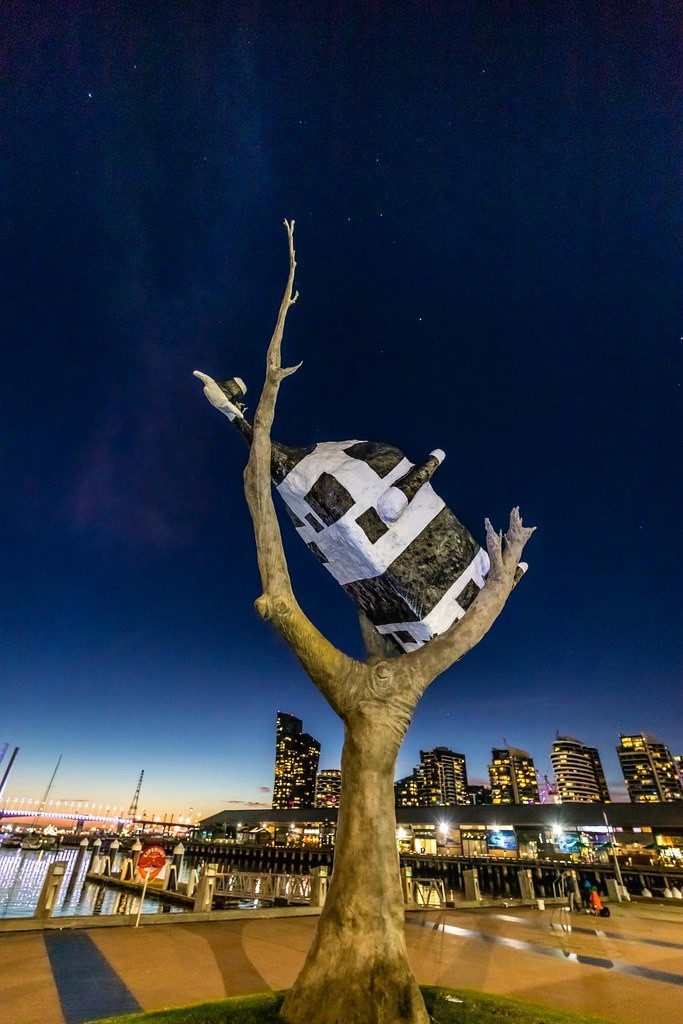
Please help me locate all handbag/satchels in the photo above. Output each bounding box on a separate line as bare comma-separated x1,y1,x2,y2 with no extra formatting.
598,907,610,917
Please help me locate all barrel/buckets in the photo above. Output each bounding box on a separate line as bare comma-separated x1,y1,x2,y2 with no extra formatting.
537,899,545,910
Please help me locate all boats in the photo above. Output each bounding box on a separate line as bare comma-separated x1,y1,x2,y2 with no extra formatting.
20,831,43,850
2,833,26,848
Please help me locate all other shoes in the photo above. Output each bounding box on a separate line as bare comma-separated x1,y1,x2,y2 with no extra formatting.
568,911,573,912
577,912,581,915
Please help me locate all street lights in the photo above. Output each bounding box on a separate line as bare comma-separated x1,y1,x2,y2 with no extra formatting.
541,823,563,861
485,822,499,854
